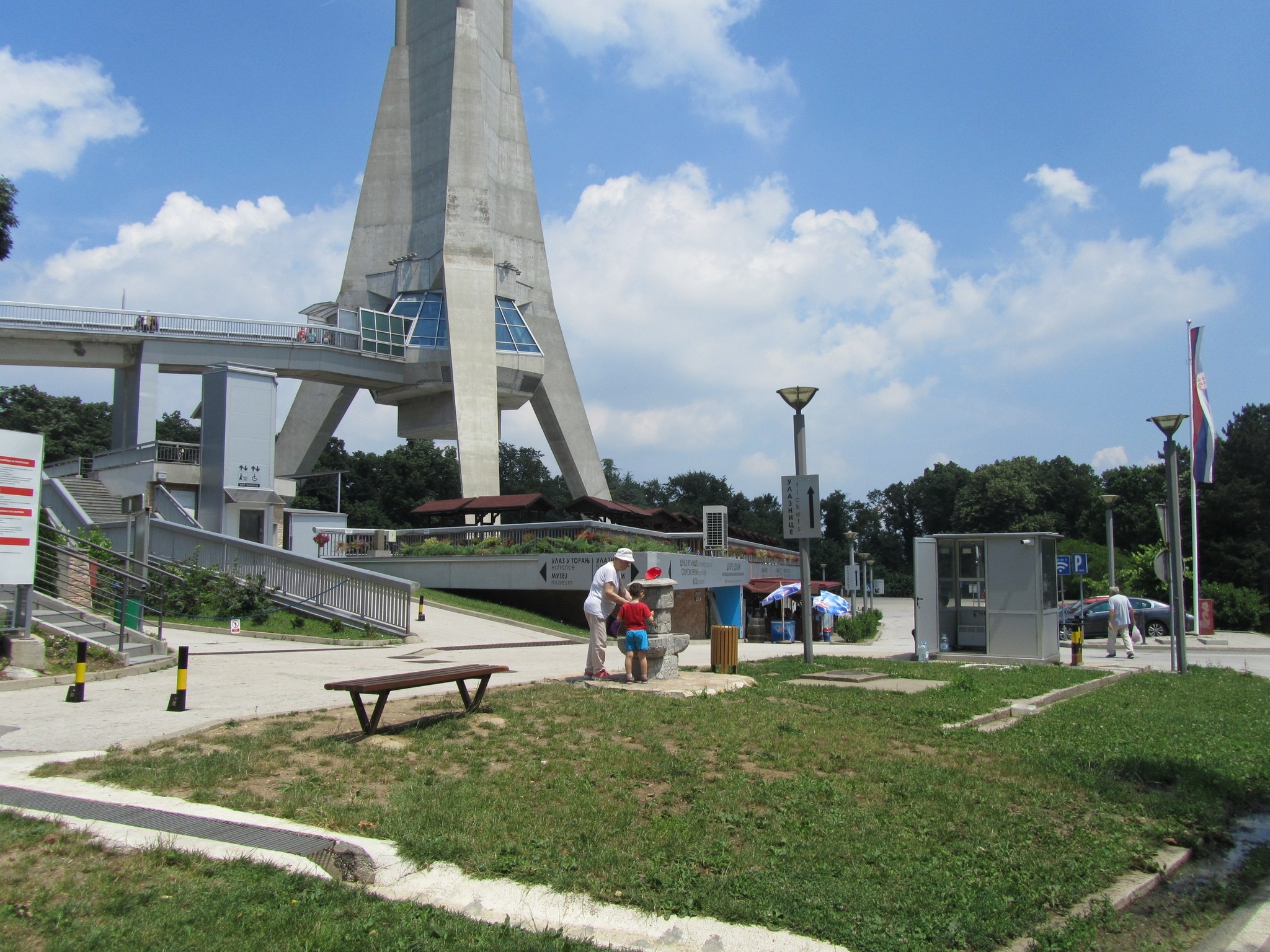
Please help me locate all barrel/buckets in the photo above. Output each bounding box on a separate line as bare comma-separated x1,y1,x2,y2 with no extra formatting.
747,618,765,643
823,630,831,641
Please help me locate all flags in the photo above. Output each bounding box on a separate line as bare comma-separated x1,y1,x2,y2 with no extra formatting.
1190,325,1214,484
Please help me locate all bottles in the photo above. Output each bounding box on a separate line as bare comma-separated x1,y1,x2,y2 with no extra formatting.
940,634,948,652
918,641,929,663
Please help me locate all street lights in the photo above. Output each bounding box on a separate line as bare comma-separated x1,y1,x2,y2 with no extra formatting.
866,560,876,611
857,553,871,613
1144,412,1188,675
842,530,859,620
775,386,821,664
1095,493,1122,589
819,564,827,581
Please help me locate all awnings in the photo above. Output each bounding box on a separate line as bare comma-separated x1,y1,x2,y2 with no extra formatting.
750,578,842,633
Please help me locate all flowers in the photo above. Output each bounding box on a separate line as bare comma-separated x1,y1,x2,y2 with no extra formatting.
313,533,330,543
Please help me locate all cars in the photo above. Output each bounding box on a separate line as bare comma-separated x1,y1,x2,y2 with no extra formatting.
1058,594,1195,641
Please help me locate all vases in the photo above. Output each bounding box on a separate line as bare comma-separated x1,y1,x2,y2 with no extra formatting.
319,543,324,547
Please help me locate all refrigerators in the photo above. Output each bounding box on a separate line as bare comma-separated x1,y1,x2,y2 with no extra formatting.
771,620,795,643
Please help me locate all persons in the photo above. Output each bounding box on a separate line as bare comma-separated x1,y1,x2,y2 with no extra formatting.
1106,586,1136,659
617,582,654,684
307,329,316,343
135,315,159,333
298,327,308,342
583,548,635,681
322,334,329,344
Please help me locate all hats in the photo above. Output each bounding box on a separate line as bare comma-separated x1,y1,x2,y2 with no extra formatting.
613,548,635,562
645,567,662,580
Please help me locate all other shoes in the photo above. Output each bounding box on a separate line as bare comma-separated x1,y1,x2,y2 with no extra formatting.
626,673,638,684
1127,653,1134,659
583,669,593,679
591,669,615,680
1106,653,1116,657
641,677,648,684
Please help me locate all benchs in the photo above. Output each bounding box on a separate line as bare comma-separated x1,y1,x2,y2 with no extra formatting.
323,664,509,732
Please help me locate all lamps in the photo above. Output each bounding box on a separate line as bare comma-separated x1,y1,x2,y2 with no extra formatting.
388,252,421,266
494,261,521,275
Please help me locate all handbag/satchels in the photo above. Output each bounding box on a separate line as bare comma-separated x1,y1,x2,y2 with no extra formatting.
606,615,621,638
1131,624,1142,644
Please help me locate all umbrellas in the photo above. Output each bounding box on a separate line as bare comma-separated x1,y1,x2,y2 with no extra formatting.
812,589,850,642
759,580,802,641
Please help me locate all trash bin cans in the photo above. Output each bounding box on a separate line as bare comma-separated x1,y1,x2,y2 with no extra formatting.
710,624,739,674
113,593,142,630
823,627,831,642
